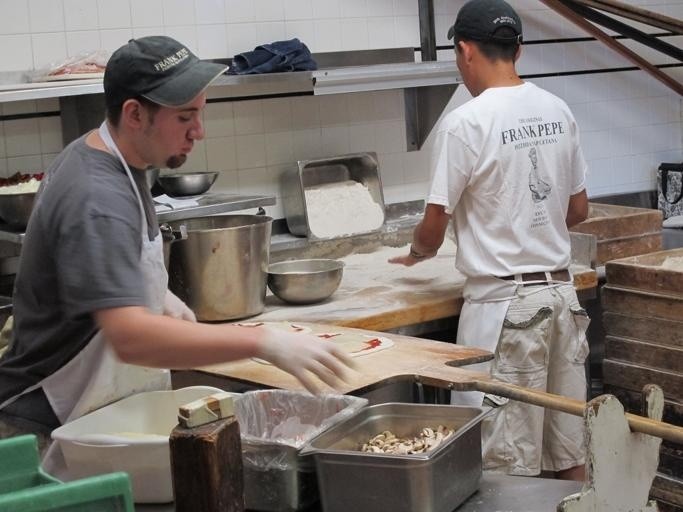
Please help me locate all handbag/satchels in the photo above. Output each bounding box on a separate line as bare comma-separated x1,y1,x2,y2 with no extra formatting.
657,162,683,219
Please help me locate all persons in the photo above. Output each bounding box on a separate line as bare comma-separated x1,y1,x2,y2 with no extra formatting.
384,0,591,485
0,34,362,466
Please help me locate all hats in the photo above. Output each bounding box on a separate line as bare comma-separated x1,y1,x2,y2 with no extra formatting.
103,35,230,110
447,0,524,45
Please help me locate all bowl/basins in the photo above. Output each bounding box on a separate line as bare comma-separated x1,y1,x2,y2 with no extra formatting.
265,257,346,305
0,191,37,230
156,170,218,199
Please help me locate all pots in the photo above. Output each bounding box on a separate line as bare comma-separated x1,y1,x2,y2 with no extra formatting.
156,206,276,324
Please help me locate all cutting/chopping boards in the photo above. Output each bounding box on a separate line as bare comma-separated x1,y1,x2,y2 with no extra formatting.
190,319,683,448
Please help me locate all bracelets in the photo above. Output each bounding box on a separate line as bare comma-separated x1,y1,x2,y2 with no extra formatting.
409,242,437,262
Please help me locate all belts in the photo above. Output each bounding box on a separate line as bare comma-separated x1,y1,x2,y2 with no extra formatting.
500,270,572,286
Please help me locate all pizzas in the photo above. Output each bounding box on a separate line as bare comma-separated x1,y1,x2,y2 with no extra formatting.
234,320,313,333
250,331,394,366
